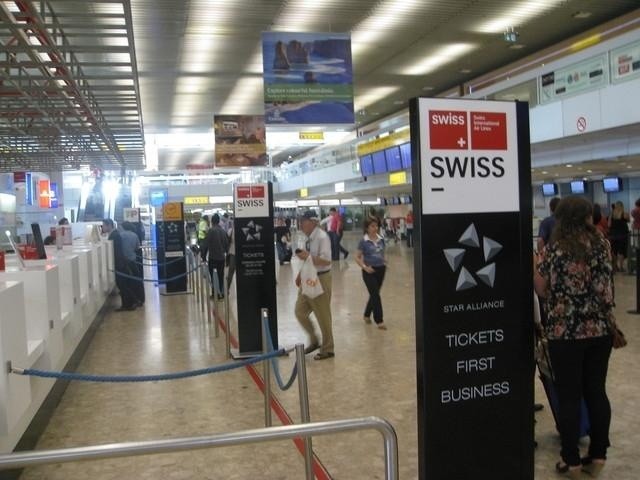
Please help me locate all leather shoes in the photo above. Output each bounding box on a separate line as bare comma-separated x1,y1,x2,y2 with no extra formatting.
114,300,142,311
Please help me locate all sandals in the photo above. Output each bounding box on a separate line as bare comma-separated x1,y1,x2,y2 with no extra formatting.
304,343,321,354
314,351,335,360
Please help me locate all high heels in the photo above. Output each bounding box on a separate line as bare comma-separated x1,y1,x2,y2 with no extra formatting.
555,461,580,479
581,456,605,478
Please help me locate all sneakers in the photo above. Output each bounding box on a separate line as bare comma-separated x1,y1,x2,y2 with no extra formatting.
363,315,373,324
378,322,386,329
343,250,348,258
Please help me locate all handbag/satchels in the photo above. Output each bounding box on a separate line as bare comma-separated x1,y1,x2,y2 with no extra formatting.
611,324,627,348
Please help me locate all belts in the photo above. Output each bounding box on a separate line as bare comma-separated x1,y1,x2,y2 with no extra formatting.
317,269,332,275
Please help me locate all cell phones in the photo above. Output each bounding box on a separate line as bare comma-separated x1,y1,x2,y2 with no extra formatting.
295,248,301,254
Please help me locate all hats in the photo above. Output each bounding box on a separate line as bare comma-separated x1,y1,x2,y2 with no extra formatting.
301,209,319,219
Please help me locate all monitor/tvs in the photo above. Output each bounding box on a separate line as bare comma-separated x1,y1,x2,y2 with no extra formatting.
602,176,623,193
380,195,411,207
542,183,558,195
569,180,586,195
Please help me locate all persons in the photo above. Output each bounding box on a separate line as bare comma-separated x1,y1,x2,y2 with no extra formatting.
45,236,57,245
59,218,69,225
275,219,293,266
406,211,413,248
102,218,146,311
319,208,349,262
533,197,640,480
294,211,337,362
357,209,390,330
193,212,236,301
